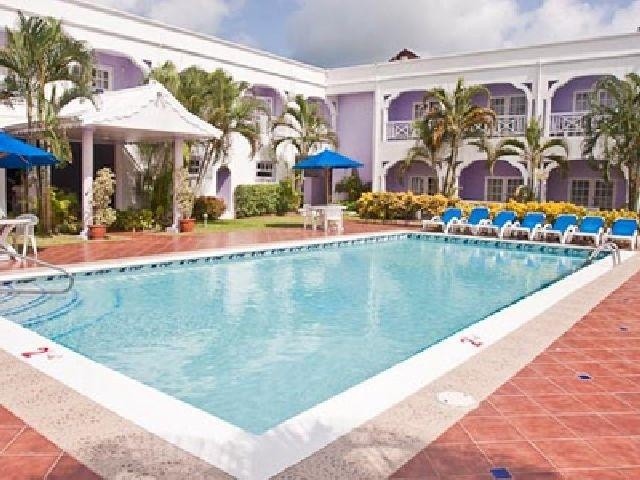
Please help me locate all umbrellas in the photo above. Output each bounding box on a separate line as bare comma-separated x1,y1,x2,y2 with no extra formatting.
0,131,58,169
292,147,364,204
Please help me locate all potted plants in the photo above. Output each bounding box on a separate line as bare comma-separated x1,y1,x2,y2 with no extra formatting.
85,166,117,241
173,166,198,233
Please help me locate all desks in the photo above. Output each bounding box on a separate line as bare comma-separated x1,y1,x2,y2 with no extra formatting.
309,206,347,230
0,219,32,262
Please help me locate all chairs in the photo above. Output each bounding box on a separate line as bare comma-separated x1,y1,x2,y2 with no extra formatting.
417,205,639,252
8,212,40,260
300,202,343,235
